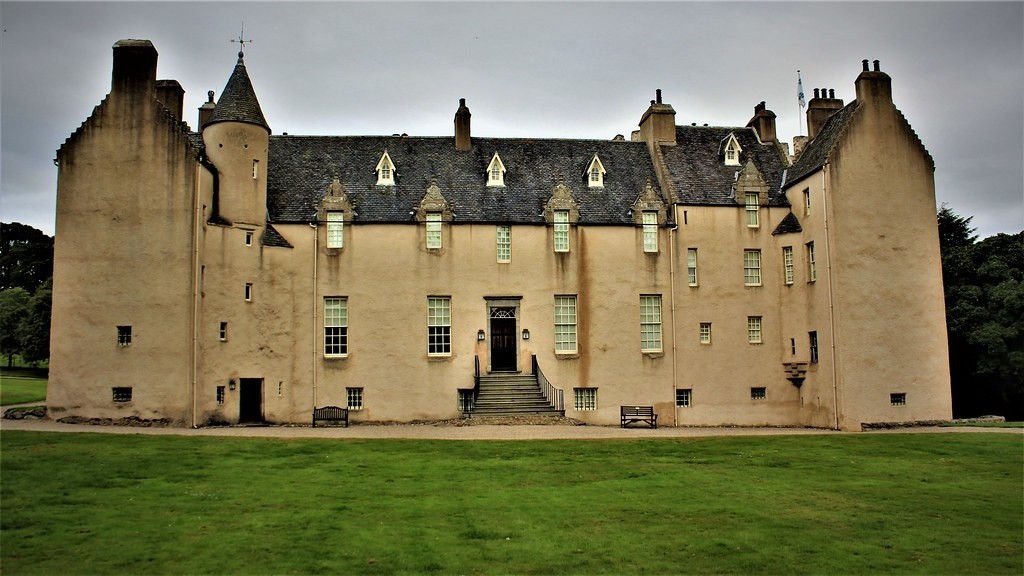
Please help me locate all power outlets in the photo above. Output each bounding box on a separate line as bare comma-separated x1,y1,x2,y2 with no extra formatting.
478,330,485,340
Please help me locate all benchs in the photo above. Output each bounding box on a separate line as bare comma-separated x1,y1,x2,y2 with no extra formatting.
311,405,349,427
619,405,658,430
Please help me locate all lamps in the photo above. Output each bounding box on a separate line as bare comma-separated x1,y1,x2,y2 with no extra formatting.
522,329,529,339
